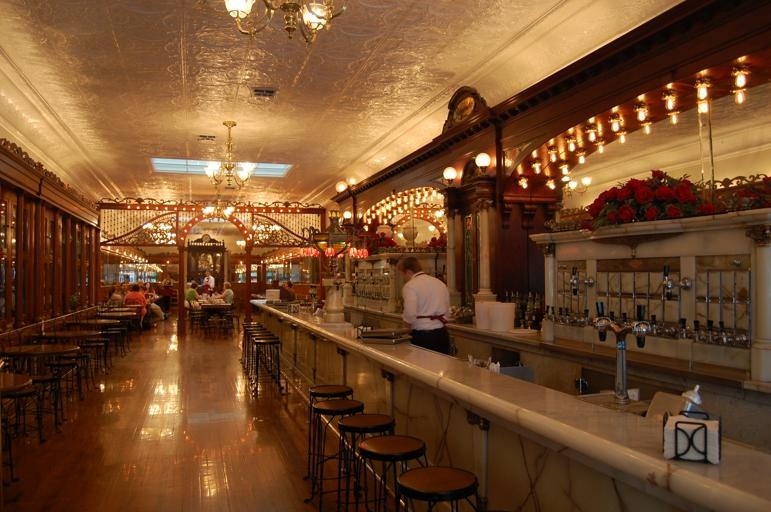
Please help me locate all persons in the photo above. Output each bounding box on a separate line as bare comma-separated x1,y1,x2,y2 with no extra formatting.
109,281,164,328
184,282,201,311
284,280,296,299
397,256,451,355
201,268,215,297
216,281,234,305
